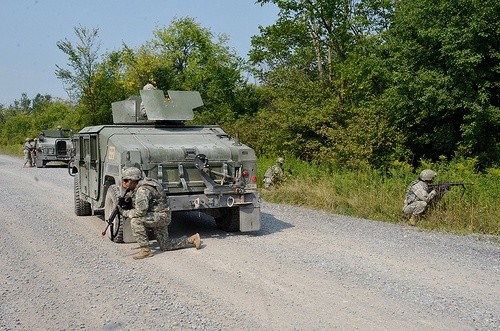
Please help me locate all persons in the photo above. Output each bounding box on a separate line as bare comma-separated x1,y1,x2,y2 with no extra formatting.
117,167,200,259
263,158,284,188
402,169,442,226
23,138,37,168
140,84,156,118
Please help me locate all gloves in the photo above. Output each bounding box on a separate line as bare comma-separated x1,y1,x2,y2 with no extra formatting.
432,190,438,196
116,205,125,215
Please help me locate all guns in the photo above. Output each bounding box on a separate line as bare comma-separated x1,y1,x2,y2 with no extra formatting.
102,188,131,236
427,181,474,196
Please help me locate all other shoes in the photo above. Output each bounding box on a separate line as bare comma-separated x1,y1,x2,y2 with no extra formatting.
409,215,416,226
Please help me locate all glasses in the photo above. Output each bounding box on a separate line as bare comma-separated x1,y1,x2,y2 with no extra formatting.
124,179,129,183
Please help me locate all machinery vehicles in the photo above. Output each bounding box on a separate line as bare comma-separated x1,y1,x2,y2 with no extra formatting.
68,89,262,243
32,129,74,168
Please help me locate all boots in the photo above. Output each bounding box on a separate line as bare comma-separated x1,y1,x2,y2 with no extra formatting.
133,249,153,260
187,232,202,249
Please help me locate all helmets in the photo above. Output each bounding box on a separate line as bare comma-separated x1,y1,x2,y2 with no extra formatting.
123,167,141,180
419,169,435,181
277,157,284,164
26,138,30,141
